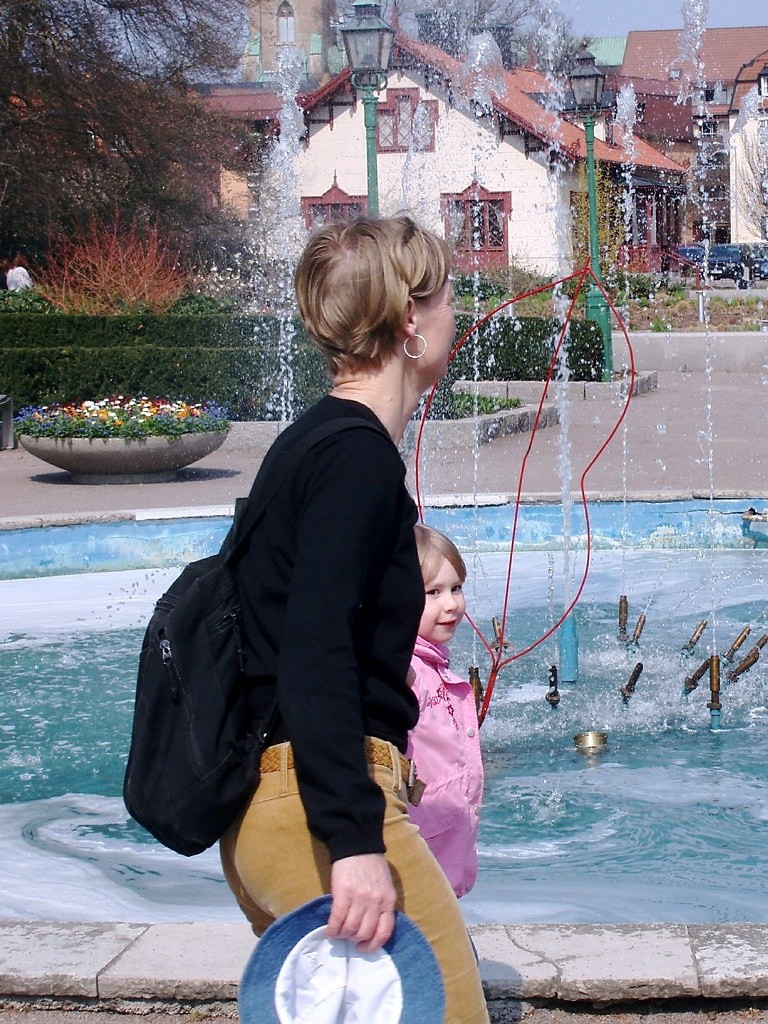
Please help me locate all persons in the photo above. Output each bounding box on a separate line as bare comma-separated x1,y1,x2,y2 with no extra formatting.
218,214,489,1023
400,523,483,963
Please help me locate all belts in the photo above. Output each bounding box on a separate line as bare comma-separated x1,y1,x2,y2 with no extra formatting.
260,742,431,807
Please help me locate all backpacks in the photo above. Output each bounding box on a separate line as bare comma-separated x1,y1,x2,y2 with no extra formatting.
119,416,389,859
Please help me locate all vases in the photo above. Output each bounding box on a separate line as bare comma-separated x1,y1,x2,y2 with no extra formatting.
18,432,229,484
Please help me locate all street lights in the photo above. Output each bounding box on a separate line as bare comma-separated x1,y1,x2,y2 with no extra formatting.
340,0,398,220
559,39,618,381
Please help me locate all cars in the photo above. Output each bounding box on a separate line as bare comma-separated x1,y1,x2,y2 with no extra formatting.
662,246,705,278
749,241,768,280
703,243,758,289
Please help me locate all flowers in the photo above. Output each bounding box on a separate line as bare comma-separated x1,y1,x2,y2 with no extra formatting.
11,393,232,444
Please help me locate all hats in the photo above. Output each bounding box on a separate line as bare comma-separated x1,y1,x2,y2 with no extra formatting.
238,893,445,1024
